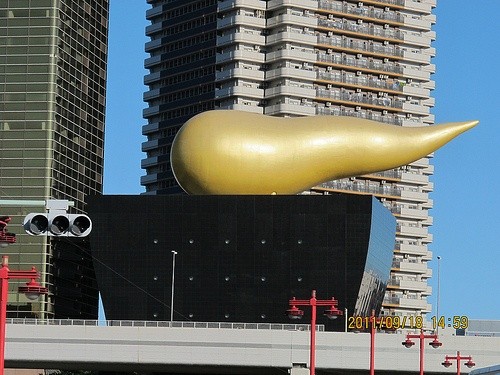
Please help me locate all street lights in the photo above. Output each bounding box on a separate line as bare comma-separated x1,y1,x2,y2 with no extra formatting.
0,254,49,375
401,328,442,375
286,290,343,375
351,310,398,375
440,352,475,375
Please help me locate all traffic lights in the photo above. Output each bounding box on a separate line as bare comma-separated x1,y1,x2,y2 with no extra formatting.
23,212,91,238
